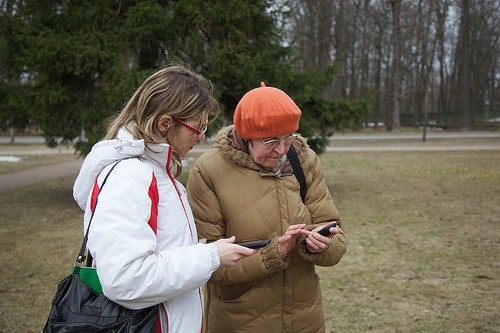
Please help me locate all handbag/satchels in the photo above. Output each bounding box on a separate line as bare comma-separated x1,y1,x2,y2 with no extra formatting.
43,267,159,333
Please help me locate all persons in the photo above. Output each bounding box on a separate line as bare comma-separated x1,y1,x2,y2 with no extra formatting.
186,80,348,333
43,64,258,333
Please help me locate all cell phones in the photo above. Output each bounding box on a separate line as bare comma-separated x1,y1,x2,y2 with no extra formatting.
233,239,270,248
300,221,337,245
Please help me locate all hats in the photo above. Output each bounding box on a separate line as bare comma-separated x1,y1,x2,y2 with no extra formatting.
233,82,302,139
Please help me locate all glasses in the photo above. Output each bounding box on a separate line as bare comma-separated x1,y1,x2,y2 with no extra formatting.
169,115,208,138
260,131,298,147
